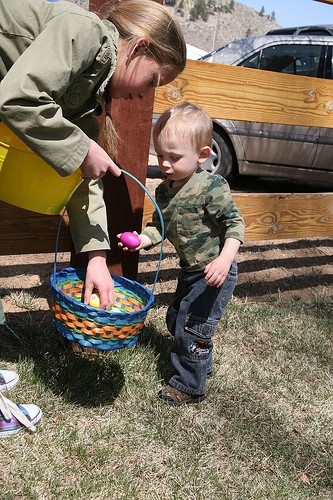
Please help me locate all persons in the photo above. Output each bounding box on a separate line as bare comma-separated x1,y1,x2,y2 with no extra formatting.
0,0,187,439
116,102,246,406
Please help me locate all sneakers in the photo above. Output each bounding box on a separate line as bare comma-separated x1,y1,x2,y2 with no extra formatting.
0,370,20,393
0,392,43,439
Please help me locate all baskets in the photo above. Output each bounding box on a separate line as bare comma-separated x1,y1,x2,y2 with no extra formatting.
49,169,164,350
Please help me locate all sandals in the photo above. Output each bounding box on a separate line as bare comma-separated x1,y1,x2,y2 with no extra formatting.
154,384,205,406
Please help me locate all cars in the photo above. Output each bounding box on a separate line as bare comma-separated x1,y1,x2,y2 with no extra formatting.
149,24,333,188
